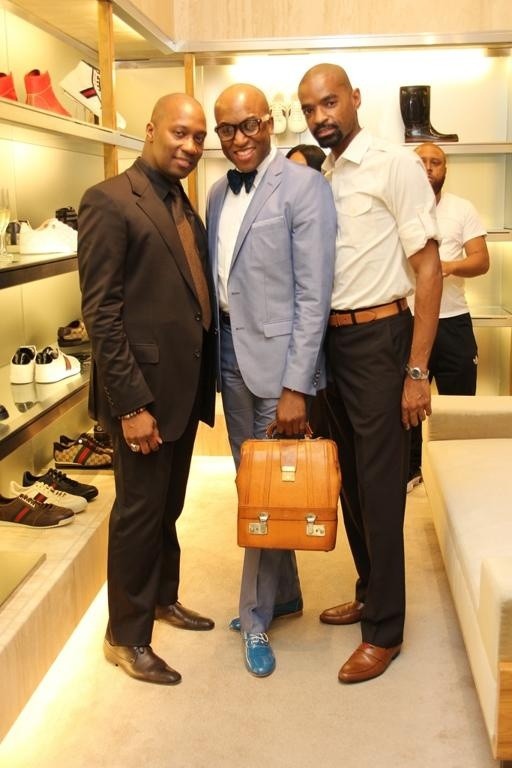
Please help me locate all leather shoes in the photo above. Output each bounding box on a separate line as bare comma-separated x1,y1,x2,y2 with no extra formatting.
338,642,401,682
103,634,181,684
244,630,275,675
319,598,364,624
231,596,304,630
154,603,214,631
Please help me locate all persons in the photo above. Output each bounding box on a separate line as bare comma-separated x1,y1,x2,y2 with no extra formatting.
409,141,490,394
201,84,341,681
293,60,446,685
76,93,219,685
281,144,330,171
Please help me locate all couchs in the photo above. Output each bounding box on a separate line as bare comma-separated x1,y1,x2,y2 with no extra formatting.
421,395,512,768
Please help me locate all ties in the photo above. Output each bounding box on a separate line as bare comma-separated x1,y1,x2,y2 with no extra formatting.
171,186,212,332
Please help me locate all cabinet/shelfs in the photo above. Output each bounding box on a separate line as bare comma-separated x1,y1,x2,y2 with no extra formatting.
0,0,200,768
177,29,512,457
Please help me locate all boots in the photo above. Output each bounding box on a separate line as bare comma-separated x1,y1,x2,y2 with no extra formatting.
399,87,458,143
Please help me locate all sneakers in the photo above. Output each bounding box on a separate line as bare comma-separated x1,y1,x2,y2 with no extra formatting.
270,93,307,133
9,320,92,385
0,73,21,101
53,424,113,468
61,60,126,129
2,204,77,255
25,68,70,115
1,470,98,528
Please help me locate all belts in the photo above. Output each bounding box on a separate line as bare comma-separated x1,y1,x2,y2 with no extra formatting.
329,297,409,328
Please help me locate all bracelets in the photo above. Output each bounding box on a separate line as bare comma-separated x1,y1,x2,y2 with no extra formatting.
118,406,147,419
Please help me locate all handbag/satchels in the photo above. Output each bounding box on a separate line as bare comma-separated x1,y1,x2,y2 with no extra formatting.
237,438,340,552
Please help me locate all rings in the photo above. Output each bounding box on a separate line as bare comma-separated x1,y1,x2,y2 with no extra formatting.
129,443,139,452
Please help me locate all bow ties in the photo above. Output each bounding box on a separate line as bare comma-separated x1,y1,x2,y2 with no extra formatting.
227,169,259,195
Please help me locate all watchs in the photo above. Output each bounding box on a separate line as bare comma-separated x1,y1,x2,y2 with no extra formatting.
404,364,430,380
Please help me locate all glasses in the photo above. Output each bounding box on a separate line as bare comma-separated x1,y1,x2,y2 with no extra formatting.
216,114,271,142
